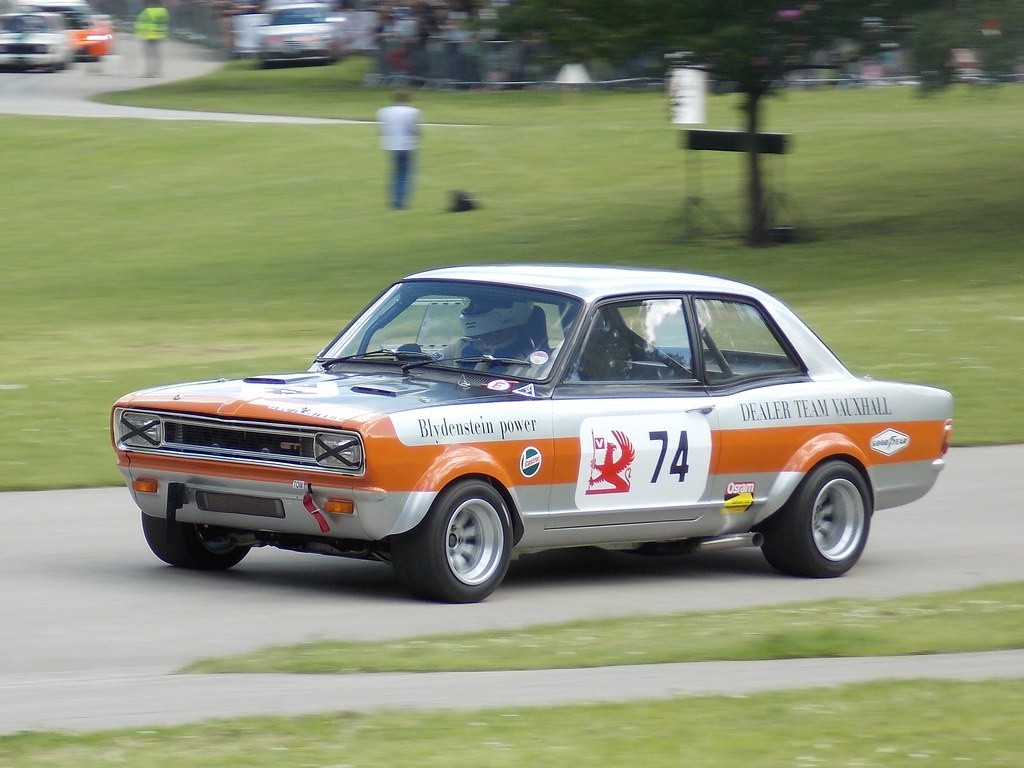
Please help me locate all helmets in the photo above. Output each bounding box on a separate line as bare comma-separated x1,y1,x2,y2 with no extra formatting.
458,286,534,338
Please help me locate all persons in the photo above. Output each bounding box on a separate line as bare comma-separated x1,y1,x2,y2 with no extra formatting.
397,296,535,377
135,1,168,75
376,93,423,207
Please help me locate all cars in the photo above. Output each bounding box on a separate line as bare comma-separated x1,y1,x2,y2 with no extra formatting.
0,2,114,74
110,264,955,603
255,3,351,70
